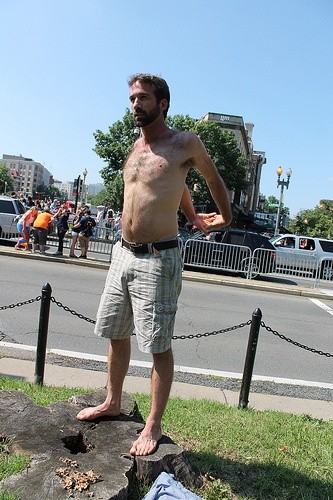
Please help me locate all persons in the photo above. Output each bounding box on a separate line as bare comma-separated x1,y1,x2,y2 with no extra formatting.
2,191,122,258
76,72,233,455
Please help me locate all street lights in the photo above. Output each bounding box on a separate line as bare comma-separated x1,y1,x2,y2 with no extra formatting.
271,166,292,236
73,168,88,214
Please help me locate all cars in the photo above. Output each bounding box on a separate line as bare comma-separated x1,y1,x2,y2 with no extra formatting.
267,234,333,281
178,228,278,280
0,196,27,239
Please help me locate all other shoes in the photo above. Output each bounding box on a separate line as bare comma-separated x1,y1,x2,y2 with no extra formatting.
31,249,35,254
70,254,78,258
54,251,63,255
40,250,46,254
79,254,87,258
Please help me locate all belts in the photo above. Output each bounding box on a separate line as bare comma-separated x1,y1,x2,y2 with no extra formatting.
118,234,178,253
